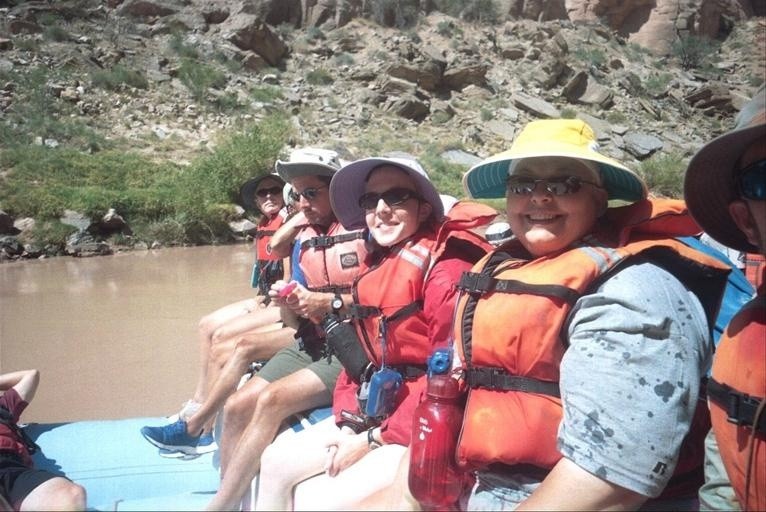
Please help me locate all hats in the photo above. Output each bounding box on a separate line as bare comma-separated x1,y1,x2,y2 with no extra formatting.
274,149,341,184
463,120,648,204
684,88,766,255
240,173,286,210
329,151,444,231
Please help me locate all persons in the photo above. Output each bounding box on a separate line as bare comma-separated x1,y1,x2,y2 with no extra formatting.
684,83,765,512
138,175,298,459
205,148,365,512
358,118,711,512
257,151,492,512
1,369,86,511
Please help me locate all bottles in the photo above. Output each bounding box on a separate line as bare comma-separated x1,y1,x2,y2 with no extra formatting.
280,280,324,325
319,313,374,385
409,375,463,509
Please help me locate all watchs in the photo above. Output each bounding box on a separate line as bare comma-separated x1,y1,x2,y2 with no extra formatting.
332,293,343,314
368,426,381,450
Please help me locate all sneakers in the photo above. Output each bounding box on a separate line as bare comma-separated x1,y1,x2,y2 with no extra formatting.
159,427,218,458
140,419,204,455
169,400,202,423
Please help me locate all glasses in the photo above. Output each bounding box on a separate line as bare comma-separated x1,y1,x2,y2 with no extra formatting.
256,187,282,197
506,175,602,196
359,188,428,209
737,158,766,201
289,185,328,201
